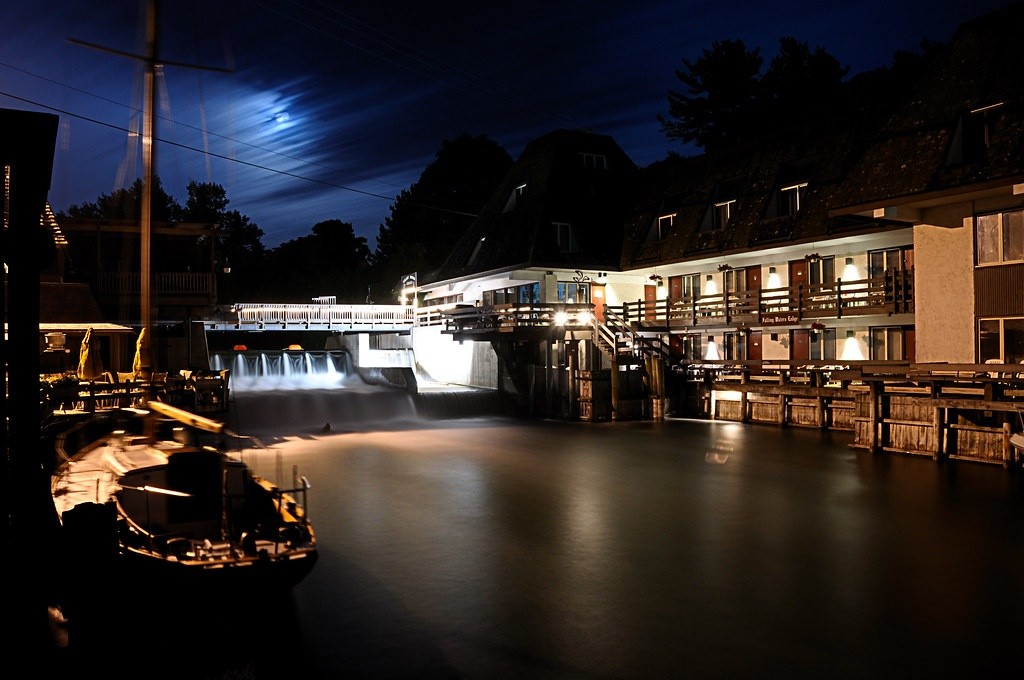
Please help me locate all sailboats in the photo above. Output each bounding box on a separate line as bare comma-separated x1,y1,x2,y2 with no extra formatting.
48,0,319,582
